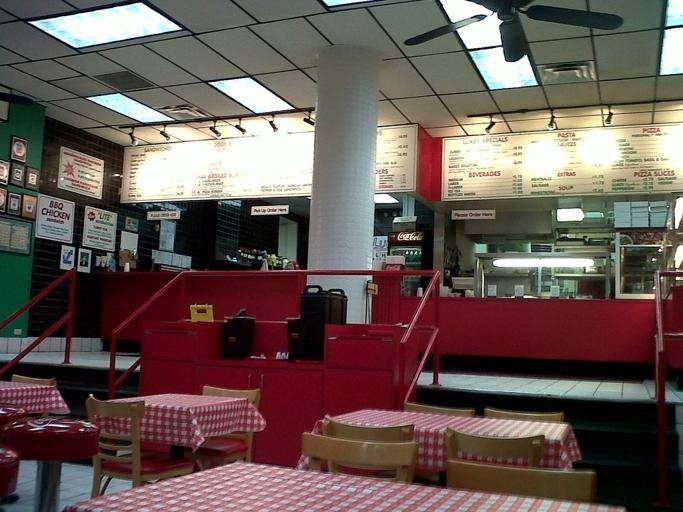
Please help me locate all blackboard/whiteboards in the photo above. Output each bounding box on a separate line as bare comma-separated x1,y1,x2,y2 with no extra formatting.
120,124,419,203
441,122,683,202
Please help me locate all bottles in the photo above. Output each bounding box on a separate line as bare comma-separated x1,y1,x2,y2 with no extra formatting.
267,254,288,269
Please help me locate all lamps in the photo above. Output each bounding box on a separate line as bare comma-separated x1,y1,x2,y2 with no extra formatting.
119,105,316,143
465,98,683,132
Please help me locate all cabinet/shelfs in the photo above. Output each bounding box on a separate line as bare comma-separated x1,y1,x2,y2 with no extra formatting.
193,364,324,474
138,320,224,453
325,322,419,475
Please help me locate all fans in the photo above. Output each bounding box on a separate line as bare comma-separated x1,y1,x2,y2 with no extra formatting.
403,1,625,63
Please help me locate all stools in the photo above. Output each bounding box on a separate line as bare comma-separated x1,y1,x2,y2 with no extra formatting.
1,418,101,511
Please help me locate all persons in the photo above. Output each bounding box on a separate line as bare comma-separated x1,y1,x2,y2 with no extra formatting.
80,256,88,267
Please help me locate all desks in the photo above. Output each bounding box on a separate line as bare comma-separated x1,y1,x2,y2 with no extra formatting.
294,409,584,488
1,380,72,418
85,392,197,500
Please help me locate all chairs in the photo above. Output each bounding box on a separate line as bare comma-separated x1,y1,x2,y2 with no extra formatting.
444,425,546,468
12,373,58,418
484,408,564,422
403,400,476,418
324,413,415,484
91,392,266,499
171,386,262,471
298,431,419,484
445,459,598,502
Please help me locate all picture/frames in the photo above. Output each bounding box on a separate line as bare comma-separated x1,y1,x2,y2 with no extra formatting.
0,135,40,255
